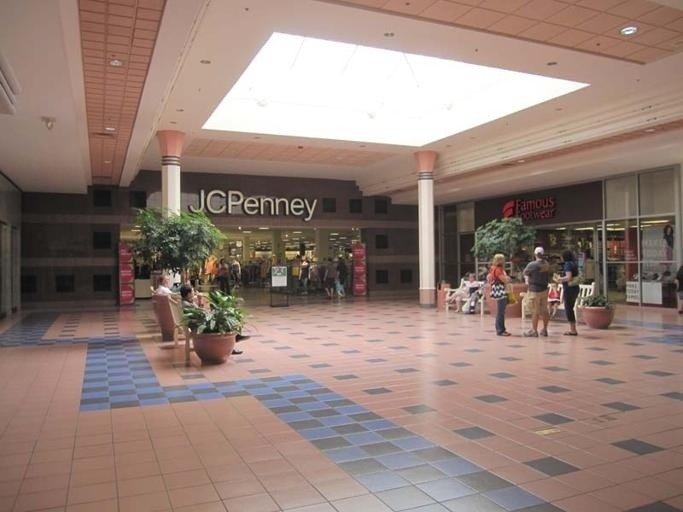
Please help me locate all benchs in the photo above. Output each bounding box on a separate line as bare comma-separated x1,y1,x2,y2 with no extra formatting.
540,282,598,322
444,278,487,316
168,298,196,367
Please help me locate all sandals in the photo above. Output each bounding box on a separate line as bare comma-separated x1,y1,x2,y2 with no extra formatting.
498,331,510,336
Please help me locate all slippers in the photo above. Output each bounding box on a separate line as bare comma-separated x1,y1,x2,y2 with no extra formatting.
563,331,578,336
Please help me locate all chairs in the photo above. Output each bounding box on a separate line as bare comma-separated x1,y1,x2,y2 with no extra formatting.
150,288,183,340
483,282,529,318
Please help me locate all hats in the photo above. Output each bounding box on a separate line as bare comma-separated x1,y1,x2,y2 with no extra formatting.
534,246,544,255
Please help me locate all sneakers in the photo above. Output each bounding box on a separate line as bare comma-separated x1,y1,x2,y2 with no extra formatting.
539,328,547,336
524,329,538,337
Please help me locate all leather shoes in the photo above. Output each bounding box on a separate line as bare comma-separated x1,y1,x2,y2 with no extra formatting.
235,333,251,342
232,347,244,354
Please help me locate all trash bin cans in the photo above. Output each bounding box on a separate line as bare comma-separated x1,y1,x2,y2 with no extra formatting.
437,282,451,313
197,292,210,310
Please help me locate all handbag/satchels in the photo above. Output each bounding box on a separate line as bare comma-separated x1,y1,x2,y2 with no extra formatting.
489,267,507,299
506,282,517,303
566,275,580,287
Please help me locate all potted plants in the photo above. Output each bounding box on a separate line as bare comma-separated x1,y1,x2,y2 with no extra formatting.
578,295,617,329
182,286,249,366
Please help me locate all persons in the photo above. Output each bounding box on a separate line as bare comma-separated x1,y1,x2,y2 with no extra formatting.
446,272,479,312
488,254,511,336
521,246,550,337
465,273,491,314
672,266,682,313
547,281,560,320
552,251,580,335
156,274,179,300
178,283,249,354
661,224,674,275
204,249,352,301
